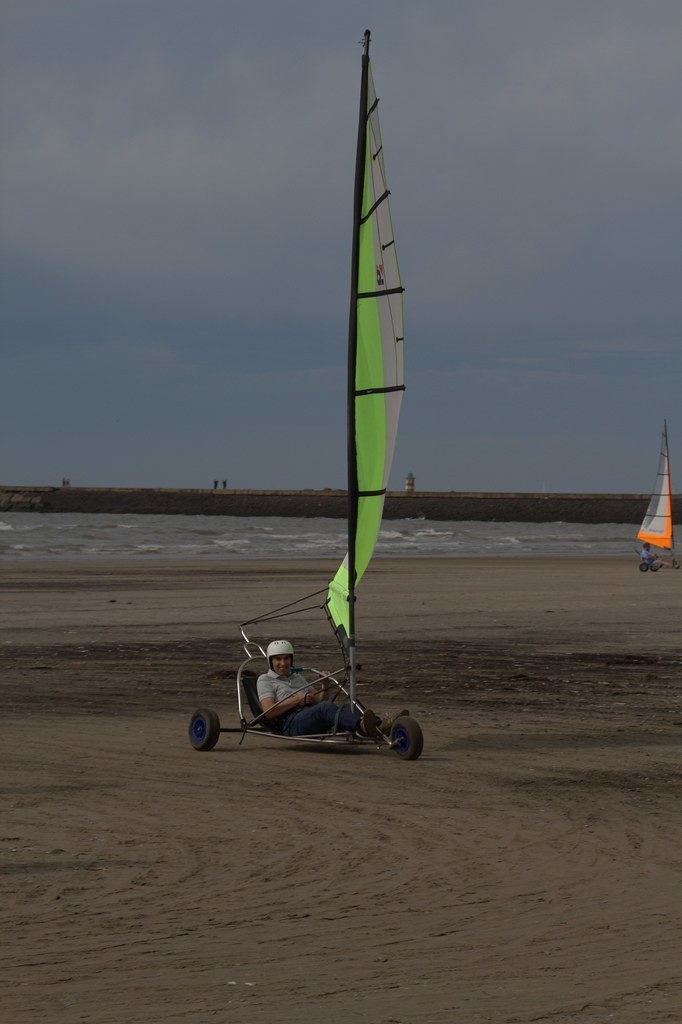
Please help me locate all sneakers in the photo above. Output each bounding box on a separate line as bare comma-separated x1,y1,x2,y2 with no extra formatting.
393,709,409,720
360,710,377,738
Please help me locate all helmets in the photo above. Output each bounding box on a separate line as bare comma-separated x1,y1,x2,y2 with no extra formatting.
267,640,297,667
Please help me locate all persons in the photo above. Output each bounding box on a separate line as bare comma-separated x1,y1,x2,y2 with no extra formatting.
255,638,410,739
222,478,228,489
213,479,219,489
67,478,70,486
641,542,679,568
62,477,66,487
